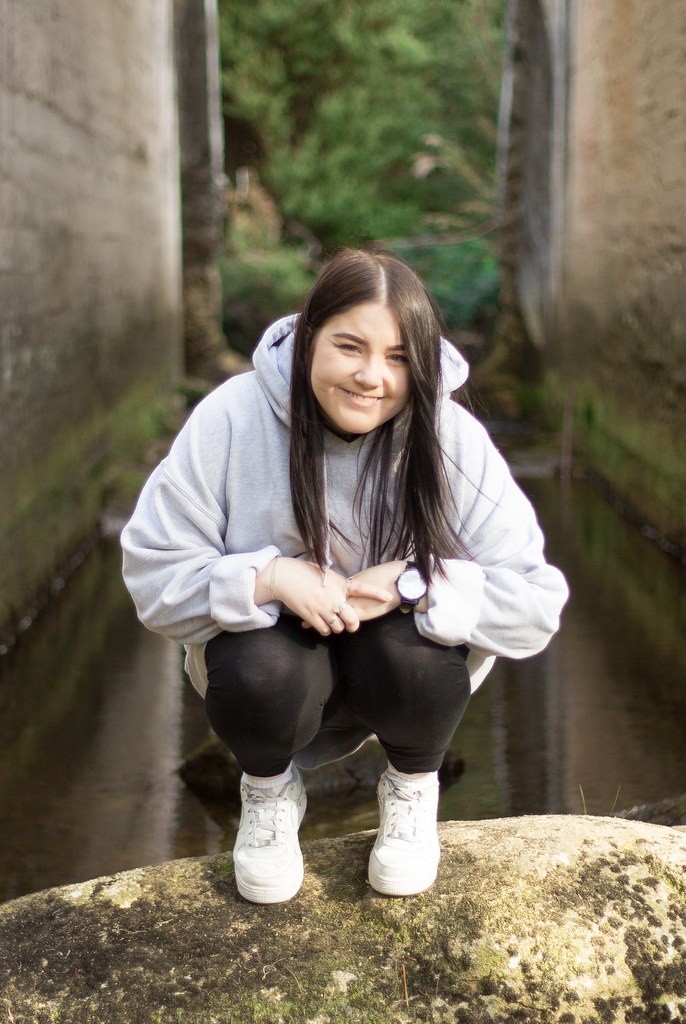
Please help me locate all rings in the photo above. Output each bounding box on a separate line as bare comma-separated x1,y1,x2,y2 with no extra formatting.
335,599,348,615
346,577,353,600
326,616,339,626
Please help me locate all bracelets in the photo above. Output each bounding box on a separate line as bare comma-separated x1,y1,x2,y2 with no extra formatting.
270,556,280,602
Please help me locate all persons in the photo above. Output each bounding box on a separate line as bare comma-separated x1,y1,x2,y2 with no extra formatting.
120,251,569,905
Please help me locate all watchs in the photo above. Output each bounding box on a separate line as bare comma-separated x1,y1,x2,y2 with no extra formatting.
395,559,429,616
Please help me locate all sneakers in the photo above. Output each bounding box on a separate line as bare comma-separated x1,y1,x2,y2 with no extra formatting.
369,767,440,895
233,760,306,904
